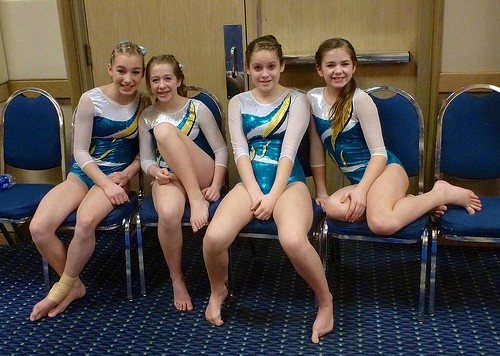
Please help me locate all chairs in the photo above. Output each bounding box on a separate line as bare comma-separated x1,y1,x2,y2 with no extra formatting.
136,86,230,295
42,106,143,301
0,87,67,249
323,86,431,314
428,83,500,316
228,86,326,290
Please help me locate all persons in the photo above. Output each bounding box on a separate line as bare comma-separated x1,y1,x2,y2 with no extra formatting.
306,38,481,236
137,55,229,311
30,41,145,321
202,35,334,344
